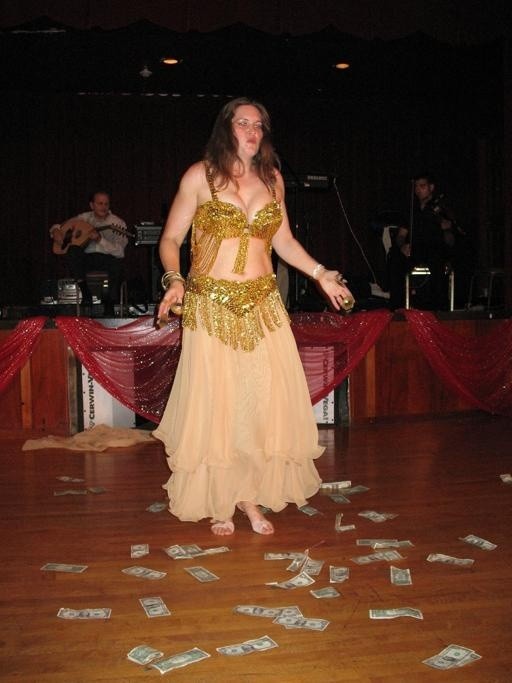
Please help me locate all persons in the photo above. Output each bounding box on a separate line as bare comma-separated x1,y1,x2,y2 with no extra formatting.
385,172,454,311
49,190,128,319
150,95,356,534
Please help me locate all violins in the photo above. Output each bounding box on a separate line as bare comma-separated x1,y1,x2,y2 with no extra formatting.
423,204,466,236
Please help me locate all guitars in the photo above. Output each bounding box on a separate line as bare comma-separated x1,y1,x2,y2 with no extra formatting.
52,221,137,255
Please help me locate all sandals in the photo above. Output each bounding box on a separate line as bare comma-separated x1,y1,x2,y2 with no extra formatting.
237,502,274,534
211,518,235,535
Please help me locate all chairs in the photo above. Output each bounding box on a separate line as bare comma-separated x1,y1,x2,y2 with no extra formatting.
382,223,456,313
74,268,129,321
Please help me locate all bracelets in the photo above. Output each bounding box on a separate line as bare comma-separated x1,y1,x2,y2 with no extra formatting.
312,263,323,278
161,270,186,292
